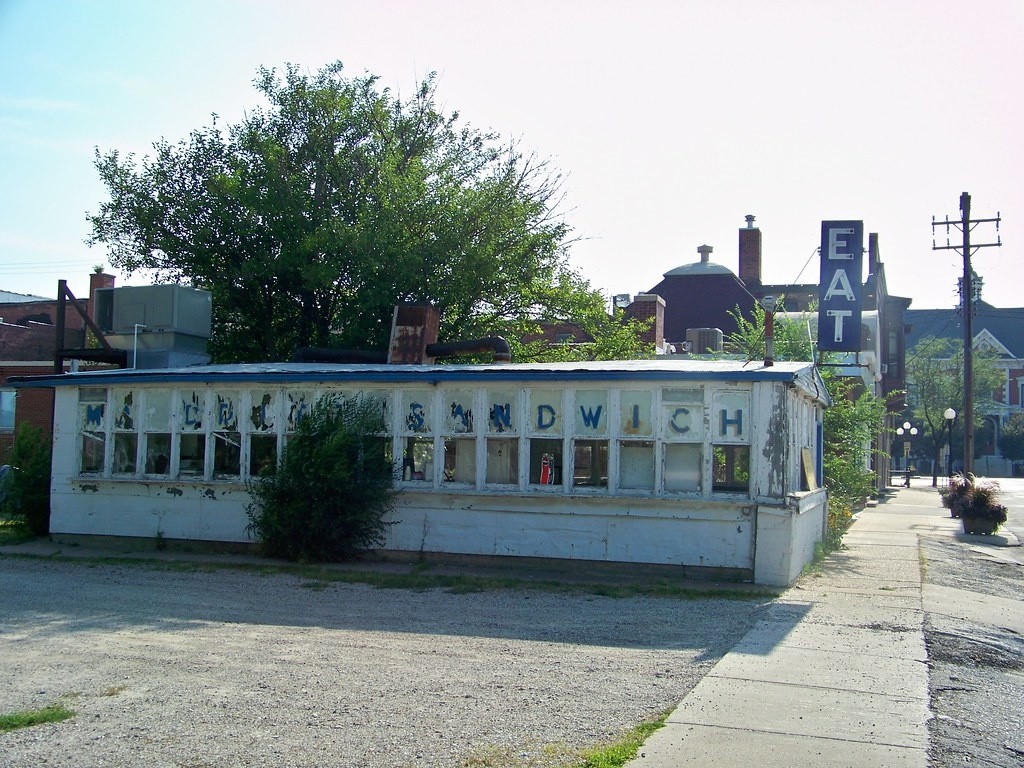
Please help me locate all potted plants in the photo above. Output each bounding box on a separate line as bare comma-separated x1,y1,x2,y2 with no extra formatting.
961,478,1010,536
942,472,977,519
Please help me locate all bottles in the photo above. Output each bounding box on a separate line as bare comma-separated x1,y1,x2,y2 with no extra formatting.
404,465,411,481
